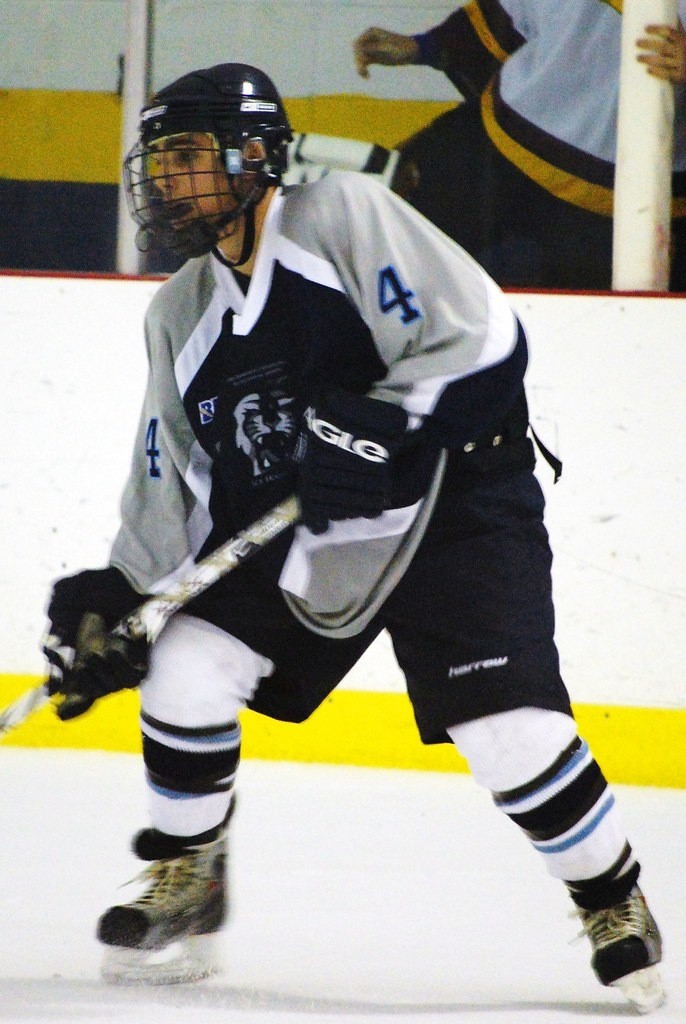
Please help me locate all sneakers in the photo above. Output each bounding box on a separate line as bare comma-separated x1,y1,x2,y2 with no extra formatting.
572,860,668,1016
98,828,228,986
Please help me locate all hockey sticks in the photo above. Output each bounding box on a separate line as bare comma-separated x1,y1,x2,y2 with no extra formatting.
0,491,301,739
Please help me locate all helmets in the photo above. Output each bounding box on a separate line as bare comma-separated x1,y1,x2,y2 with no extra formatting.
121,62,295,257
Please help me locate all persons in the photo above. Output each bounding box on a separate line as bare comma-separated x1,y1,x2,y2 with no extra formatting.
276,0,686,293
41,62,666,1016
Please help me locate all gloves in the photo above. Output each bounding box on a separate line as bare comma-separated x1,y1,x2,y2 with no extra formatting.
279,384,409,536
43,563,150,722
